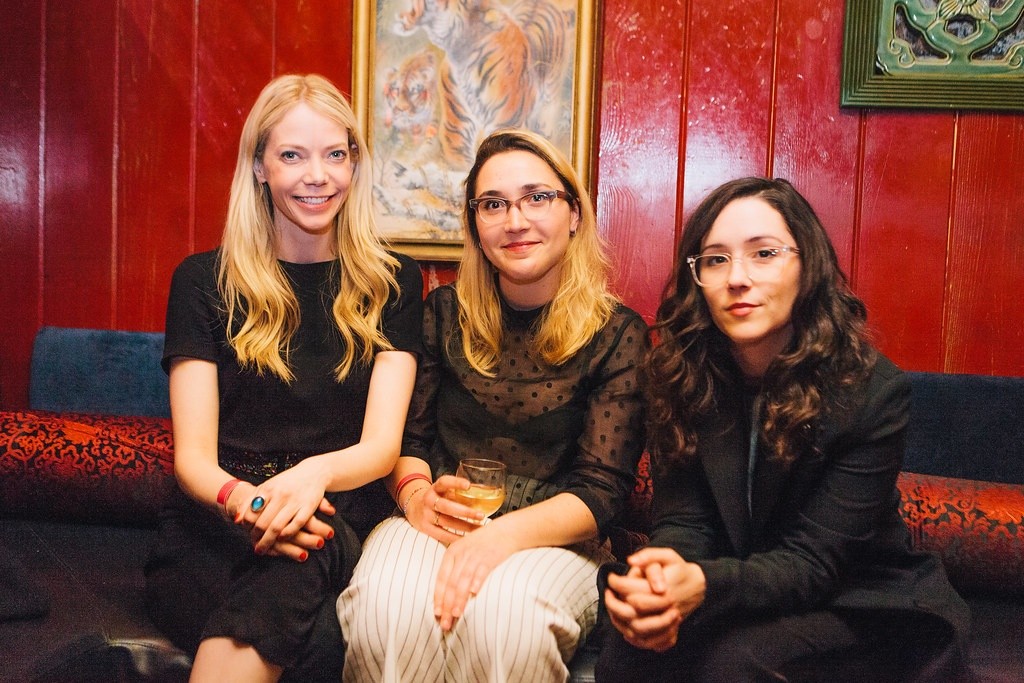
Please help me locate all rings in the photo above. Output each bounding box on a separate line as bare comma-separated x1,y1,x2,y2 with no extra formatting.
435,495,445,512
250,495,266,511
435,514,441,524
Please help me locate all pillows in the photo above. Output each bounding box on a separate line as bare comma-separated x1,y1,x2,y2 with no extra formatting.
624,450,653,523
892,471,1024,576
0,411,189,517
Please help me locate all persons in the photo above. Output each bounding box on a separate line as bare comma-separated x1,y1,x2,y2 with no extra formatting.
335,130,650,682
591,177,957,681
161,75,423,681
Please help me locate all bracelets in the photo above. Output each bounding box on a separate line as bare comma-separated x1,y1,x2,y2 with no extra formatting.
395,474,432,522
216,477,245,525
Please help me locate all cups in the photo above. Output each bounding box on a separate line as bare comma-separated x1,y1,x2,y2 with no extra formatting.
453,458,508,525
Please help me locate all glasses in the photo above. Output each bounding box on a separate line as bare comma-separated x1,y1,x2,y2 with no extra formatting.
469,190,575,221
684,245,805,288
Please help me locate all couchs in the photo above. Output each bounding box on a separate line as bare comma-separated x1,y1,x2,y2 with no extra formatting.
0,330,1024,683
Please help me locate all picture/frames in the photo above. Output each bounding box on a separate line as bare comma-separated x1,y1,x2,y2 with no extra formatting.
351,0,605,264
839,0,1024,112
